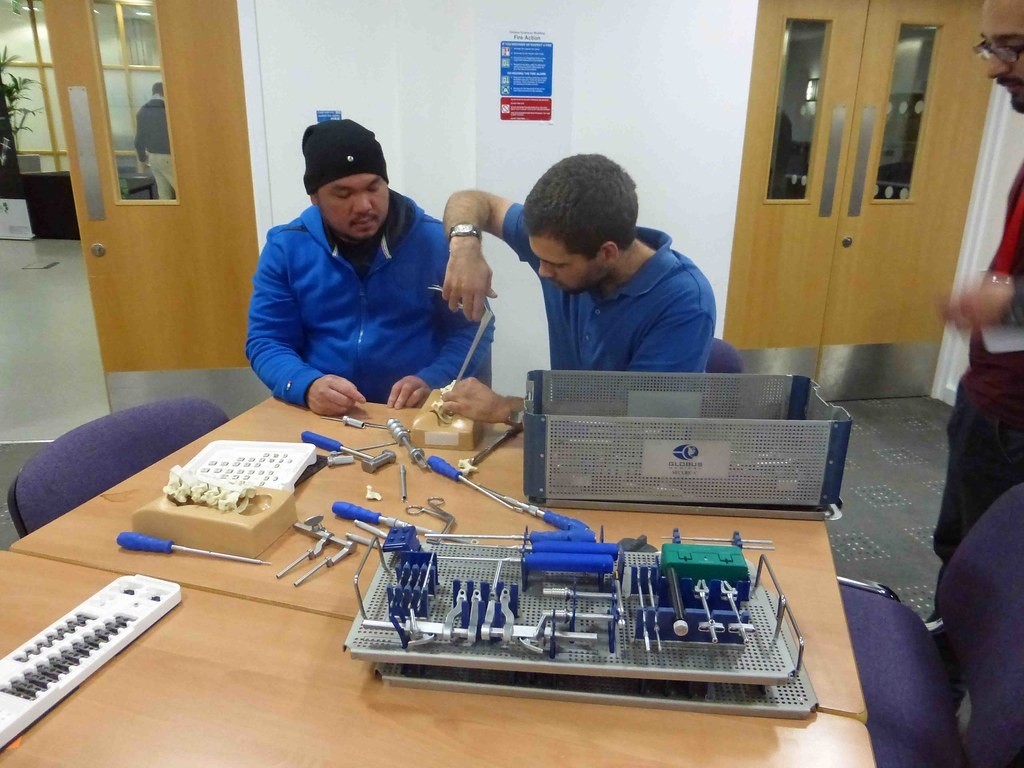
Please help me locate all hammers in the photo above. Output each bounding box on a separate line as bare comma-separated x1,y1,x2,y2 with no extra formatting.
301,430,396,474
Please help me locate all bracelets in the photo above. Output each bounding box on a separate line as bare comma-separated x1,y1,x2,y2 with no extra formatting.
994,276,1012,283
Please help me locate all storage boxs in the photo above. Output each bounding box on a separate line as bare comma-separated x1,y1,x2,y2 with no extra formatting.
523,369,855,520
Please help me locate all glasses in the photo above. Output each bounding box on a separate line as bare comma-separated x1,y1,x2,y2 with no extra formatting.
972,39,1024,63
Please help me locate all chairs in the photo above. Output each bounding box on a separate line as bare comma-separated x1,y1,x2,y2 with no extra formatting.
838,481,1024,767
7,398,229,538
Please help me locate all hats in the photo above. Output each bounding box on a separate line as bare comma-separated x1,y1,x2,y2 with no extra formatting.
301,118,389,195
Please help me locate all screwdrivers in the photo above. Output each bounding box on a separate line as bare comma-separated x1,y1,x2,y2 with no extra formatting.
332,501,472,544
117,531,273,566
423,530,620,574
427,456,523,513
477,483,591,530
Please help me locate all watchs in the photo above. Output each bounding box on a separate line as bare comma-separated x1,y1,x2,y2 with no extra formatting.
447,222,482,242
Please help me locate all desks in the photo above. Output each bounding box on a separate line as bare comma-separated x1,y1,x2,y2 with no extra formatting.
0,396,875,768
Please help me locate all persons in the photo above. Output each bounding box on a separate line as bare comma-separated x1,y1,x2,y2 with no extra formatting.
133,82,178,199
441,152,715,424
244,121,495,417
927,0,1024,619
